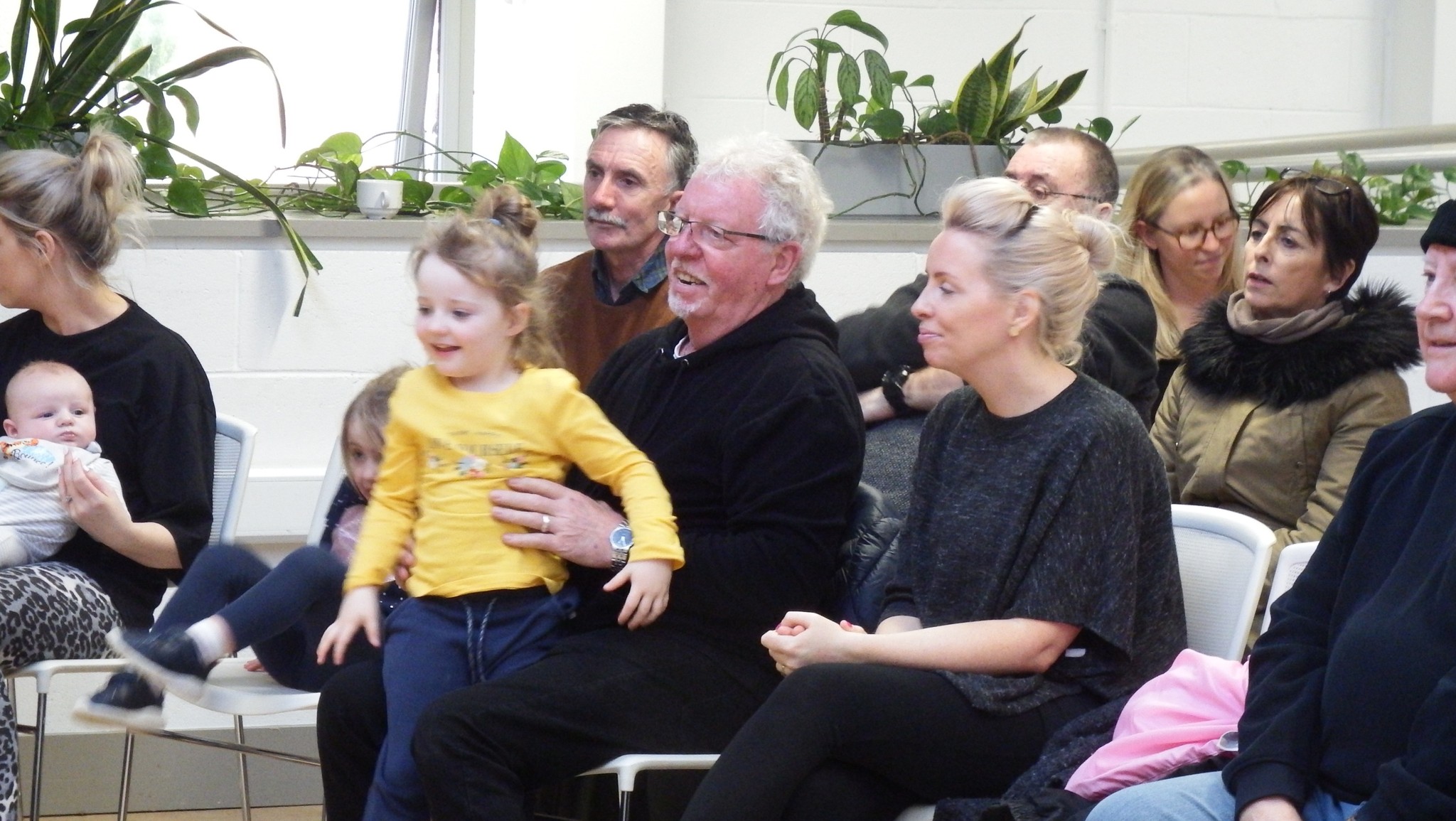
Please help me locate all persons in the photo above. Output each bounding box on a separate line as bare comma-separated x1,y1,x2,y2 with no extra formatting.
0,126,217,821
0,360,130,569
314,103,1456,821
71,363,415,728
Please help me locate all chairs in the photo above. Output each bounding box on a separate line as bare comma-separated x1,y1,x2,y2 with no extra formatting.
7,412,249,821
573,479,883,821
898,504,1278,821
119,434,349,821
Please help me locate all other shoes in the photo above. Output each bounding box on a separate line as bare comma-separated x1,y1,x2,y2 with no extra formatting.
74,673,170,731
106,623,217,701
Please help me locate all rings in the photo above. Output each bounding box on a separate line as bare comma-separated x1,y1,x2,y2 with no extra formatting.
541,513,550,532
65,495,73,503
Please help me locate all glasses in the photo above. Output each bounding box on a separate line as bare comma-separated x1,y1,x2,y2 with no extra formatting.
1279,167,1355,224
1020,184,1106,204
1146,208,1242,250
656,209,782,249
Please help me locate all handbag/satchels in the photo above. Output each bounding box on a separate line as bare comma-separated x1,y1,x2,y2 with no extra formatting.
1066,650,1252,797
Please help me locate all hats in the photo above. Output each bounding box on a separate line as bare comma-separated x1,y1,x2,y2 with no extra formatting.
1419,198,1456,255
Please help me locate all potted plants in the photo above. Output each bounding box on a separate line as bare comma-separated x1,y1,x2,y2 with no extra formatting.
767,10,1456,227
0,0,590,317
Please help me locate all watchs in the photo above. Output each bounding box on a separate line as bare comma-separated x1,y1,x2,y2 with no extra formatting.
881,364,912,415
609,519,635,572
